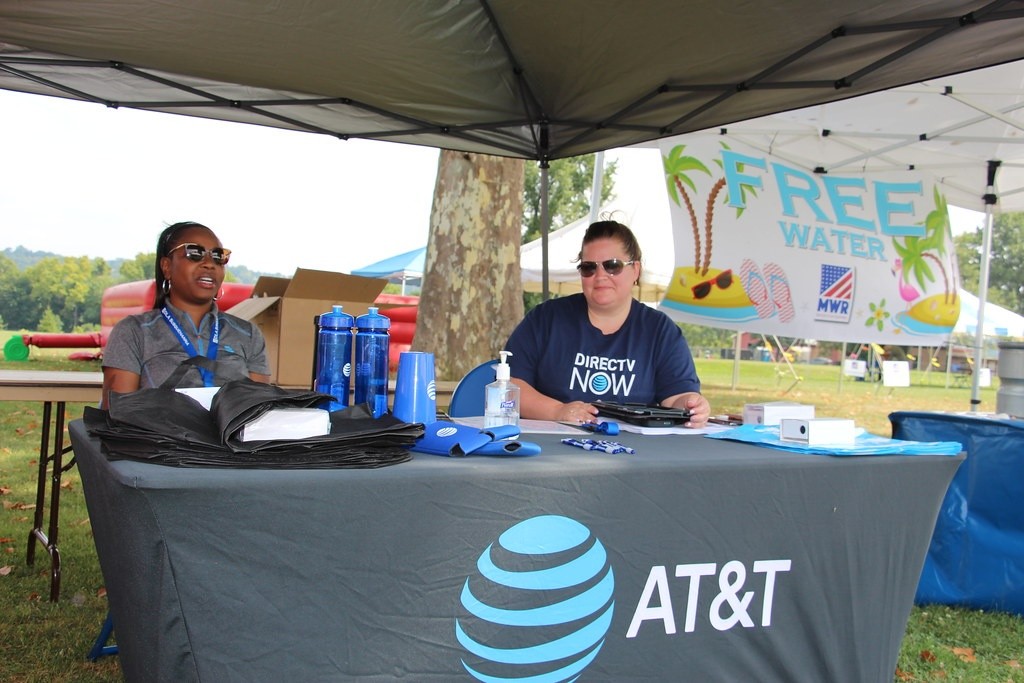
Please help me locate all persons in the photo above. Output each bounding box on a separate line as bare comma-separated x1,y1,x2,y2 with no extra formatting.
101,222,271,411
503,221,710,428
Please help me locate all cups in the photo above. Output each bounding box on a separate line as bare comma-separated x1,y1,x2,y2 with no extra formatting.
393,351,438,425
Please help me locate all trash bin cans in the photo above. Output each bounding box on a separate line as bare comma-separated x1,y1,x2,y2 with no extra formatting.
762,350,770,361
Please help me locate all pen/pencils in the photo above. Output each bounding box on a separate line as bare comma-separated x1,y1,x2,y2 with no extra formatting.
561,438,636,454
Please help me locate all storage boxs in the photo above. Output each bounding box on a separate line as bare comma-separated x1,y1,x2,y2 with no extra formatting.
222,267,420,390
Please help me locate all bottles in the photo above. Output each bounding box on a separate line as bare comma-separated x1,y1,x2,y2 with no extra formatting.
355,307,392,418
314,305,355,413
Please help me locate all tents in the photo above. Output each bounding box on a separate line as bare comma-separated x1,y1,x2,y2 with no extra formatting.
352,246,426,296
518,199,675,307
590,55,1024,416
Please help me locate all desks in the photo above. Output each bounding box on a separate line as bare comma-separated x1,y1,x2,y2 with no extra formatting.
68,416,968,683
0,369,104,605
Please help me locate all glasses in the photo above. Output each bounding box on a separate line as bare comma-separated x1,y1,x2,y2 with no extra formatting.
577,259,634,277
167,243,231,264
